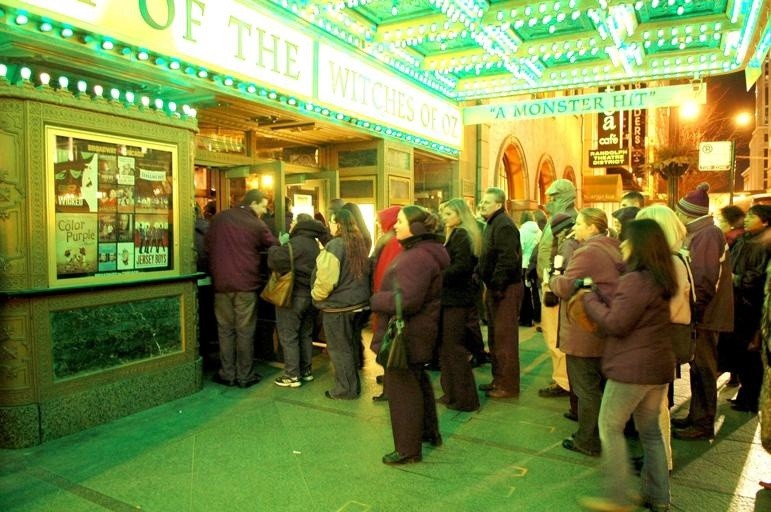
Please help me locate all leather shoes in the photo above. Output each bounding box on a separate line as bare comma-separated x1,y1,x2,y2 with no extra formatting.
670,418,688,428
373,393,387,401
383,450,421,464
539,382,583,451
239,374,261,388
671,429,713,441
479,382,495,391
486,389,519,398
422,432,442,446
215,374,237,386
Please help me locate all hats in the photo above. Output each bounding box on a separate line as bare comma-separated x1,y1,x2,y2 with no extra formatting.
550,212,574,235
675,182,709,217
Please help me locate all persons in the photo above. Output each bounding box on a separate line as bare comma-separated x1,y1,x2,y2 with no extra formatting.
195,187,524,412
513,177,770,512
370,204,452,465
99,160,166,254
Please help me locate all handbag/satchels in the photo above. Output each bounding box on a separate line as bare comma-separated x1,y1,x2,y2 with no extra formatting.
670,252,697,364
376,289,410,375
260,241,295,308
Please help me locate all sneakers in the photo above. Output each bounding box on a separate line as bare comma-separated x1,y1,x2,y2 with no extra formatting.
275,376,302,387
301,366,314,381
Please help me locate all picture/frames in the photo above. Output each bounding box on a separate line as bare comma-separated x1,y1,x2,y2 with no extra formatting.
388,175,412,206
340,174,378,204
45,124,181,287
337,147,378,170
388,145,412,172
